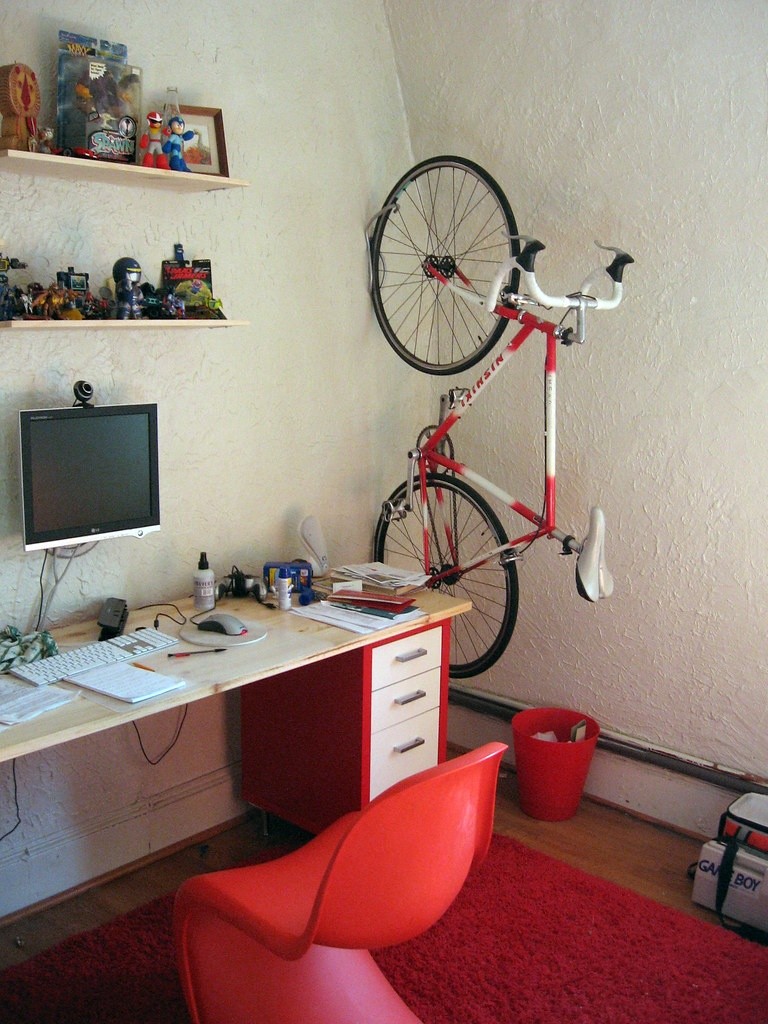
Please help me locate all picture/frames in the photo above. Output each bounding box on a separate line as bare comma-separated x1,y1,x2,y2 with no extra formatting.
166,101,233,176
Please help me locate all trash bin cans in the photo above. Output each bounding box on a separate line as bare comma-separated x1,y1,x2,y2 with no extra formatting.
511,706,601,823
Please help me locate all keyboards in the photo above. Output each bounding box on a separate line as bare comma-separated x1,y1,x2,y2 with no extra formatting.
9,628,179,687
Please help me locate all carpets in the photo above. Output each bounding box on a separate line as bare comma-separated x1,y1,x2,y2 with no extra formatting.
2,829,768,1024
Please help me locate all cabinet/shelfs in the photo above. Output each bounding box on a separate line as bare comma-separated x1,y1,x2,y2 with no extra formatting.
0,148,247,329
1,578,476,837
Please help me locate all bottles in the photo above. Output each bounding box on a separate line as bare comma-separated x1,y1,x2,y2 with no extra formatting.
277,565,292,610
193,550,215,610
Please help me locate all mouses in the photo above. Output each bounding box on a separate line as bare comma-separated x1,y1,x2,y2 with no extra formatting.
197,613,249,636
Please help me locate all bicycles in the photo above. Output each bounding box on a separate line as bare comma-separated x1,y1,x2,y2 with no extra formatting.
369,156,635,680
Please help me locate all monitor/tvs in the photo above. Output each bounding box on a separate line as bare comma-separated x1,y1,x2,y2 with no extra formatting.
18,403,161,552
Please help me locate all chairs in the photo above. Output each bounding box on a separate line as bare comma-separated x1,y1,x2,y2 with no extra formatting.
170,736,508,1020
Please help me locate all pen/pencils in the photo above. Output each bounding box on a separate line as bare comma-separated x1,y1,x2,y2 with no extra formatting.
167,648,228,658
132,660,157,672
